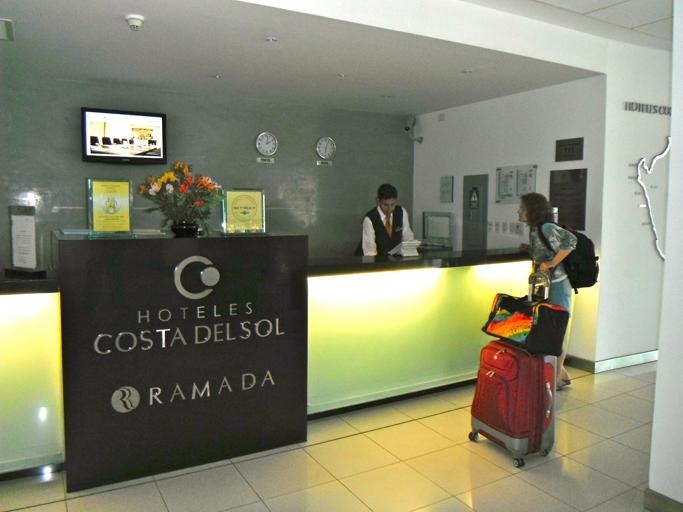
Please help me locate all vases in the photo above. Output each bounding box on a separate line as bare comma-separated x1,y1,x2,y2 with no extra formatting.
171,223,200,236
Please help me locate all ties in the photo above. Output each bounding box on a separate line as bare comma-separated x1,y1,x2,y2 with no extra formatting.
385,217,392,238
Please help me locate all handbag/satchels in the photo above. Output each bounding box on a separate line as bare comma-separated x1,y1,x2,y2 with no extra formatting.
482,294,568,356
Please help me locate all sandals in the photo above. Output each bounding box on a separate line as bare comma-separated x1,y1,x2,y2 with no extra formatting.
556,380,570,391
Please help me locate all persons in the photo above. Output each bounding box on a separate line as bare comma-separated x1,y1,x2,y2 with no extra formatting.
355,184,414,257
517,192,579,391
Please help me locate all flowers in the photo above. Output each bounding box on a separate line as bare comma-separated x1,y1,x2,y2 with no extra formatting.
137,161,225,236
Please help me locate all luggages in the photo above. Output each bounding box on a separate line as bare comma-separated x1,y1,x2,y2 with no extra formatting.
469,271,558,467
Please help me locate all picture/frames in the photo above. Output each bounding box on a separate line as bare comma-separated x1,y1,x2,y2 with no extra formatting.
222,187,266,234
87,178,137,239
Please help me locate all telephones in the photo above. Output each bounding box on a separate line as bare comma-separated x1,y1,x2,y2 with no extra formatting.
389,240,423,256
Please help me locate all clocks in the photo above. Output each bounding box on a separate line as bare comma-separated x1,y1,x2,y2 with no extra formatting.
256,132,278,156
316,136,336,160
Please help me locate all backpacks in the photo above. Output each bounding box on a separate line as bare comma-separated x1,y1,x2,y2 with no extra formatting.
538,221,599,294
